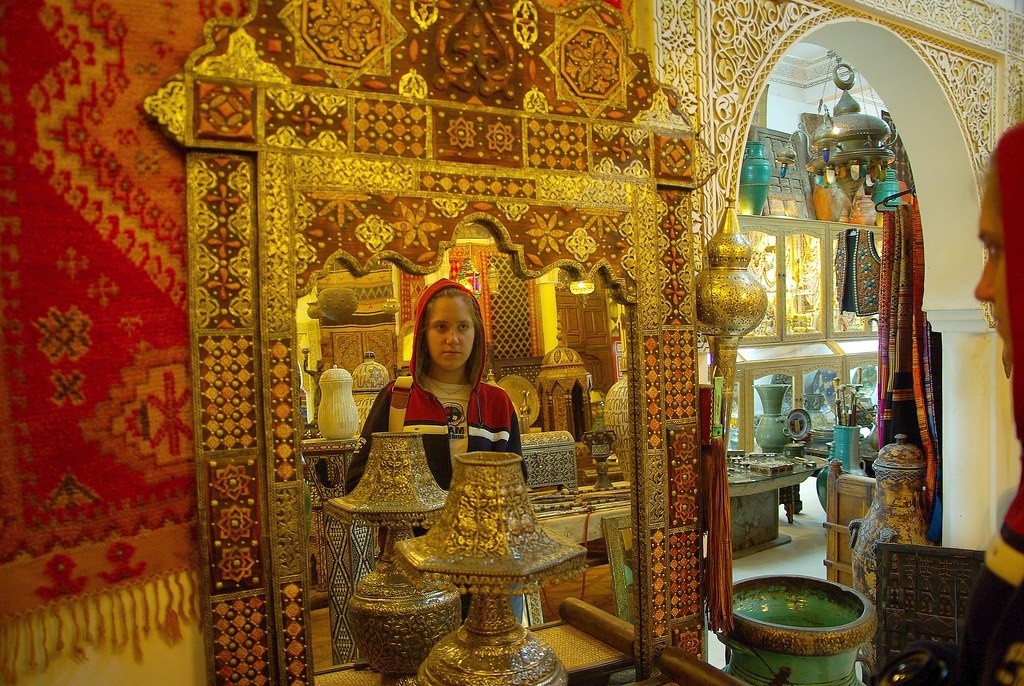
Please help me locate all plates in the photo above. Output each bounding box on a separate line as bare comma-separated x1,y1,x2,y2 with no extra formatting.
787,409,811,441
497,375,540,426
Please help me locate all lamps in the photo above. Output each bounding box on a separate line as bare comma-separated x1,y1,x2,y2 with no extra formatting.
326,431,463,686
391,450,588,686
776,51,899,186
568,273,596,310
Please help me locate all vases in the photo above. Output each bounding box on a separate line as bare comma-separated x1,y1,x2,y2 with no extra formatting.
816,423,866,514
750,383,792,454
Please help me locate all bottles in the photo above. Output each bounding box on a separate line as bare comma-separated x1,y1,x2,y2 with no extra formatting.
752,383,792,453
738,141,772,215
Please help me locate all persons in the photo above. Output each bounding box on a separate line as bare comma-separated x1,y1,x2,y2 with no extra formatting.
952,122,1024,686
344,277,528,624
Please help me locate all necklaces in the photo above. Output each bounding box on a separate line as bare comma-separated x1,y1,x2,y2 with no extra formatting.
431,376,467,395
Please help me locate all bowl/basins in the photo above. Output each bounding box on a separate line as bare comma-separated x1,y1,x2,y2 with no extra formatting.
718,571,879,654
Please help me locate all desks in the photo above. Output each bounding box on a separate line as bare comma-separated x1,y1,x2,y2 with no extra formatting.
314,595,636,686
724,456,830,563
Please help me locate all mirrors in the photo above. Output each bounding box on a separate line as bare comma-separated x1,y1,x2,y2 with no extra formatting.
143,1,722,686
